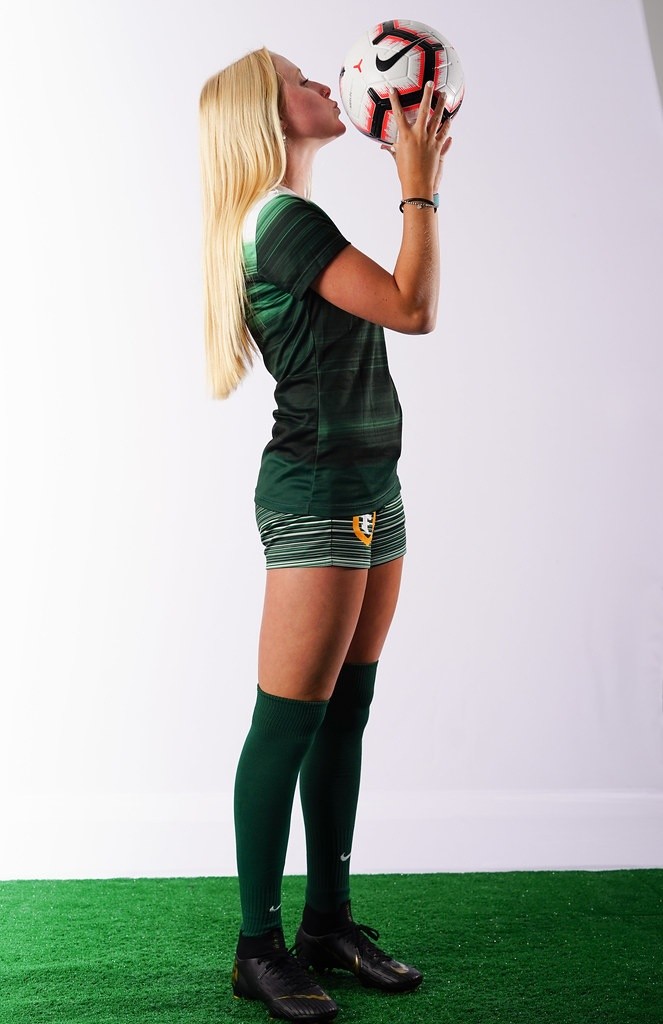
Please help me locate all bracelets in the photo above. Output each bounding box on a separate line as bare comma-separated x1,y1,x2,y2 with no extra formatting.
399,197,438,213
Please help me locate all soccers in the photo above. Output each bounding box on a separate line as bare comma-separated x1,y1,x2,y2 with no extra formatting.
337,19,465,147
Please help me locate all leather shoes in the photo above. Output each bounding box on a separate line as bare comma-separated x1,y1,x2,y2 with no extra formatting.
233,952,340,1021
298,923,423,993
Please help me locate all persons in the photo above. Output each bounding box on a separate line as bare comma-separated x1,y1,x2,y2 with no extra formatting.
194,45,456,1024
